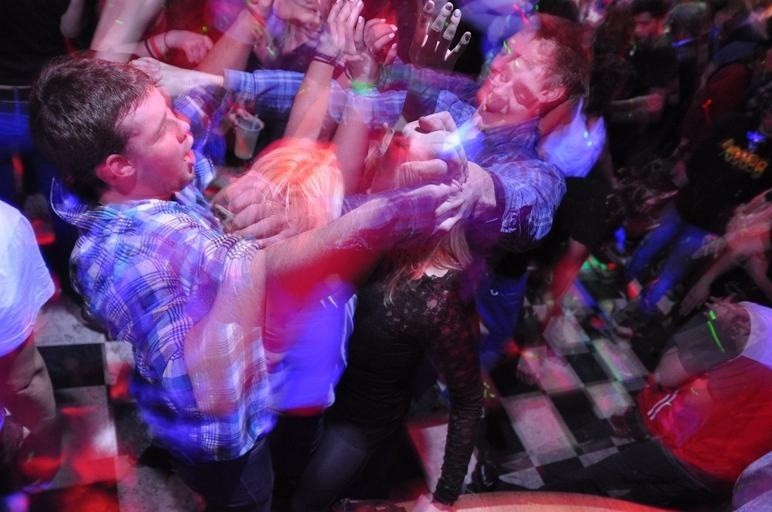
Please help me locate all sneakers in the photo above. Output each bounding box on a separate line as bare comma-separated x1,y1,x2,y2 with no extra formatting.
614,299,640,325
596,269,625,296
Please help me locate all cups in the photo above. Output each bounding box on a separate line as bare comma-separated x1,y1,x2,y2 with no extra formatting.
233,113,263,162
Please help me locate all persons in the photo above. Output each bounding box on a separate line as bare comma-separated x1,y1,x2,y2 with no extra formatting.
0,0,772,512
